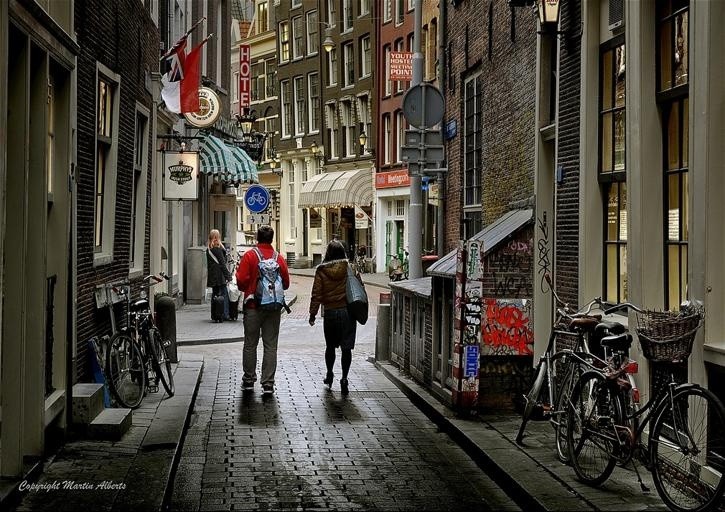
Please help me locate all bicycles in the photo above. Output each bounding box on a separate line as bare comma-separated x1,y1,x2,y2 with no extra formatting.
106,272,175,410
400,248,409,279
350,245,366,274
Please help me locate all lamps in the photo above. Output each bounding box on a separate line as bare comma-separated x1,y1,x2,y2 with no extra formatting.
269,158,283,177
237,114,255,141
322,24,335,51
359,131,373,155
311,140,325,170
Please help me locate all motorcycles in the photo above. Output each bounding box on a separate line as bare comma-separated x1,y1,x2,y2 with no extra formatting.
388,254,403,281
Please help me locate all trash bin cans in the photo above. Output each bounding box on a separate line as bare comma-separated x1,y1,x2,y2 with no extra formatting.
155,295,177,363
313,254,322,266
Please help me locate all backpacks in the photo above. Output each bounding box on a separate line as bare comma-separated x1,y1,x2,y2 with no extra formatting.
252,247,285,313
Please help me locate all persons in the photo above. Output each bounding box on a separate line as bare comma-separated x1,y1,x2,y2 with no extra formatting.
204,227,232,320
306,239,359,395
234,226,290,396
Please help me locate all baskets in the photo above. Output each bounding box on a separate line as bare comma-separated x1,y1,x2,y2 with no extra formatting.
635,306,701,361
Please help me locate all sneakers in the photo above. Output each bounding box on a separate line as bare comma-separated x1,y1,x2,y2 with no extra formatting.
222,316,232,321
262,386,273,393
242,382,253,389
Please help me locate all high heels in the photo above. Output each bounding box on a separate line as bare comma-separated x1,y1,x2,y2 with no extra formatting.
324,371,333,388
341,379,348,395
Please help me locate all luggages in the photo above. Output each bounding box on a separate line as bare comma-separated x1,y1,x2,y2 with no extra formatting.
211,282,238,323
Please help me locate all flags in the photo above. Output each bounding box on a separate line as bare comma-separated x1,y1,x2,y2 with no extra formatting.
163,21,193,80
159,45,201,113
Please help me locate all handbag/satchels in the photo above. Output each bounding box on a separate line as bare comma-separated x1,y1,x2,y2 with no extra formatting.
346,276,368,325
